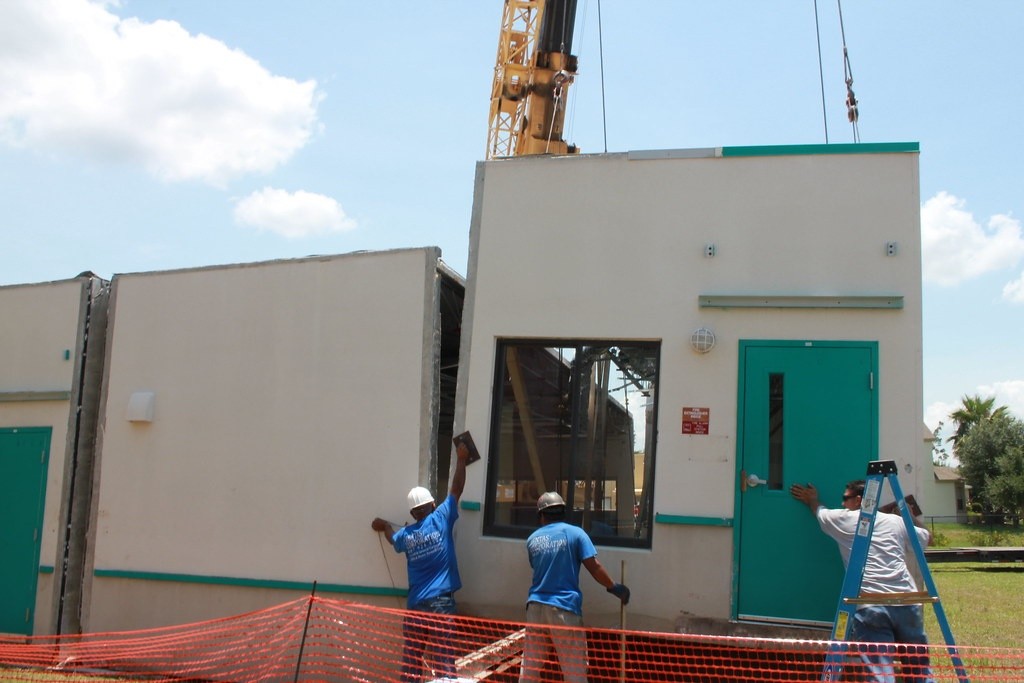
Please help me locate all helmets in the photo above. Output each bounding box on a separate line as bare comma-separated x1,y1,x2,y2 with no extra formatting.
407,486,435,511
536,491,566,513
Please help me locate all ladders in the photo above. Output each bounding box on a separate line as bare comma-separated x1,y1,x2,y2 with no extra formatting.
821,460,968,683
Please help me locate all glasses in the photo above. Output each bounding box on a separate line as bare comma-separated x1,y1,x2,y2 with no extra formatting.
842,494,860,502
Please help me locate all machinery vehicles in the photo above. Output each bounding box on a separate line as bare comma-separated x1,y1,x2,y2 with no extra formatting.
479,0,638,160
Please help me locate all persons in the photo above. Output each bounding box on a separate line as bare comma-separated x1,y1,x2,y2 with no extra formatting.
519,492,630,683
790,481,936,683
372,441,467,683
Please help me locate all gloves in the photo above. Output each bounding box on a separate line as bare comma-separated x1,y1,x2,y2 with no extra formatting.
607,583,630,605
